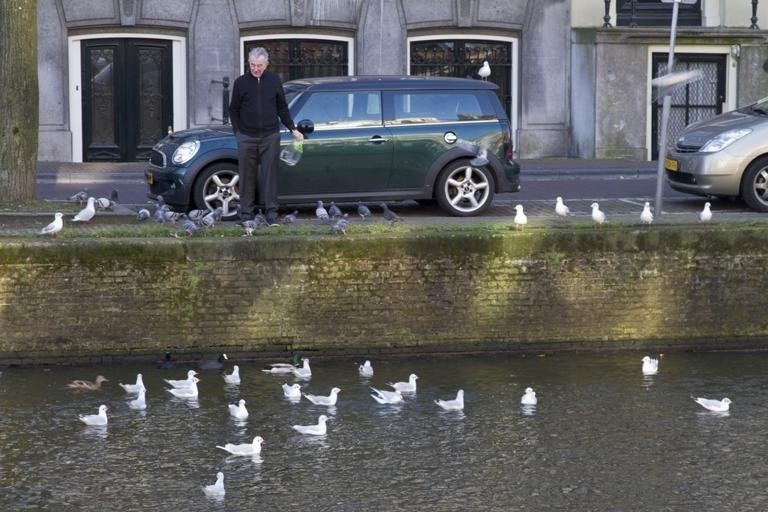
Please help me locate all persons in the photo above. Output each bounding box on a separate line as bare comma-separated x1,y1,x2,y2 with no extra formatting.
230,47,304,227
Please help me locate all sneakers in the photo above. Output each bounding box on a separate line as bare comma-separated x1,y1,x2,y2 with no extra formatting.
235,214,281,227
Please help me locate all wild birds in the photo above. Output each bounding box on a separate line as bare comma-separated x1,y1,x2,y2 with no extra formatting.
689,394,732,413
66,187,119,225
118,372,147,394
433,389,466,411
477,60,491,82
281,383,301,400
261,358,313,378
370,386,403,404
200,470,225,497
590,202,609,225
521,386,538,405
35,211,64,239
228,399,249,419
165,377,200,397
641,355,660,377
303,387,342,406
293,415,335,435
162,370,200,388
125,387,147,411
135,193,406,239
555,196,576,218
698,201,713,225
513,204,528,233
221,365,241,384
358,359,374,377
640,201,654,226
215,436,265,456
78,404,108,426
385,374,419,392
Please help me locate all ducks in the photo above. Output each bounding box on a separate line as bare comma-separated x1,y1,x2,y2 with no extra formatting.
68,375,110,391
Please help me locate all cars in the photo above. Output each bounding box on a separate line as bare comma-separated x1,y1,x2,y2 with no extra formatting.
664,96,768,214
142,71,523,221
